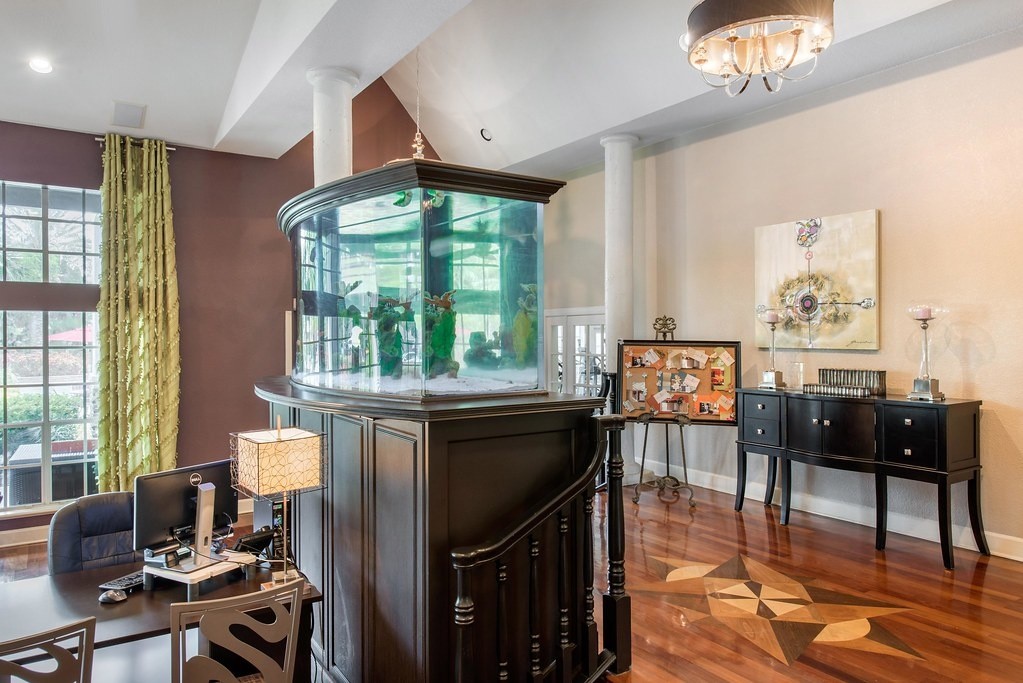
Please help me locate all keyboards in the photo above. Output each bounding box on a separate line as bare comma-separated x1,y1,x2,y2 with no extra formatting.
99,570,159,590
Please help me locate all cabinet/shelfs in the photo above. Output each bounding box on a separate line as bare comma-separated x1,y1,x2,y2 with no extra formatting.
252,373,605,682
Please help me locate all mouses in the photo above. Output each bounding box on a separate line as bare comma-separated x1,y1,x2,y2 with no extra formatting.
98,589,128,603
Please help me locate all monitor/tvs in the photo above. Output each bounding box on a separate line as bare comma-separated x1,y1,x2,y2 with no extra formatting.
132,457,238,551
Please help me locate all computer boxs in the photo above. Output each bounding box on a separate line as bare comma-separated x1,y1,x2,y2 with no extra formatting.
254,492,292,561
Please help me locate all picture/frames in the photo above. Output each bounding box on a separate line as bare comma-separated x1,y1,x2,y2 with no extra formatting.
617,339,741,428
755,210,880,348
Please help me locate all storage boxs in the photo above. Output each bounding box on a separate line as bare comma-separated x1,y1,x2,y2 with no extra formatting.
276,155,567,404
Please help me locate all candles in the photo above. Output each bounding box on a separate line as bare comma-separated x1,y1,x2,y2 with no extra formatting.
765,311,778,321
914,305,932,319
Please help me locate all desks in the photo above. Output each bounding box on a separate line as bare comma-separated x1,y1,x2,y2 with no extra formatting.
0,539,321,669
733,387,993,576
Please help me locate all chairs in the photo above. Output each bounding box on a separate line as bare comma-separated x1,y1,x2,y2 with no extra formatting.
168,578,304,682
46,491,143,576
1,615,98,683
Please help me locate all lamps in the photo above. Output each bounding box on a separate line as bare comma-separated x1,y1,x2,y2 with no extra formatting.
677,0,835,97
229,415,329,592
